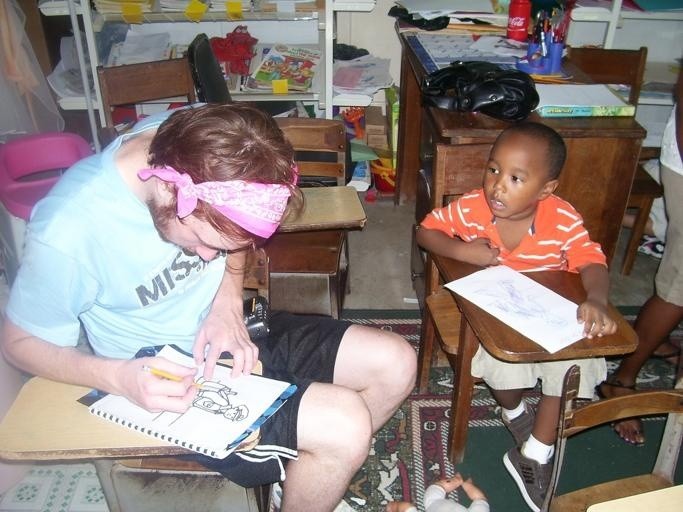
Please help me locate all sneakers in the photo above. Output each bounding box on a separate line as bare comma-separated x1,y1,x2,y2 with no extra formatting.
500,400,553,512
638,234,665,259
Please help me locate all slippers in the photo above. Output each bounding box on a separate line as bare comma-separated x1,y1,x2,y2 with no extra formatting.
596,379,647,448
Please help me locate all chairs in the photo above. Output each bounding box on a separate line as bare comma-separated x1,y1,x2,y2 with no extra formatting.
101,237,269,512
564,42,665,277
94,55,195,143
182,36,359,188
248,117,354,319
419,188,594,460
541,363,683,511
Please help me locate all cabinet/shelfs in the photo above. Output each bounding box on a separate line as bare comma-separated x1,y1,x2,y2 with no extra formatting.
519,1,681,145
39,0,377,155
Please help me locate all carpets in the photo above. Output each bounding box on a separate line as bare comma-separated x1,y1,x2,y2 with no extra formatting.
268,303,682,512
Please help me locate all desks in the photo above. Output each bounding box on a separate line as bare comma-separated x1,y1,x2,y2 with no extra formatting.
264,185,368,235
431,249,640,364
0,357,262,461
391,12,648,391
582,484,683,512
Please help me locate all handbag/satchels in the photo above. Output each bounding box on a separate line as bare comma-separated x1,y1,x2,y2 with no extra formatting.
423,62,539,123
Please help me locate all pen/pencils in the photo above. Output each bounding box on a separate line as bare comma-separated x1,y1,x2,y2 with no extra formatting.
536,7,567,57
142,365,209,391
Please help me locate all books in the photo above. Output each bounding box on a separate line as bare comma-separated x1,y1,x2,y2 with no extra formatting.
89,345,291,460
531,82,635,119
240,42,323,95
37,0,318,16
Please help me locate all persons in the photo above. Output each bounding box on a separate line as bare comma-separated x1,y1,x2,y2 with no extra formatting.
416,122,619,512
595,65,683,448
0,101,419,512
384,471,491,512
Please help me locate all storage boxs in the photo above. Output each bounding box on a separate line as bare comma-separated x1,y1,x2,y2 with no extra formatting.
383,85,399,169
363,104,388,154
